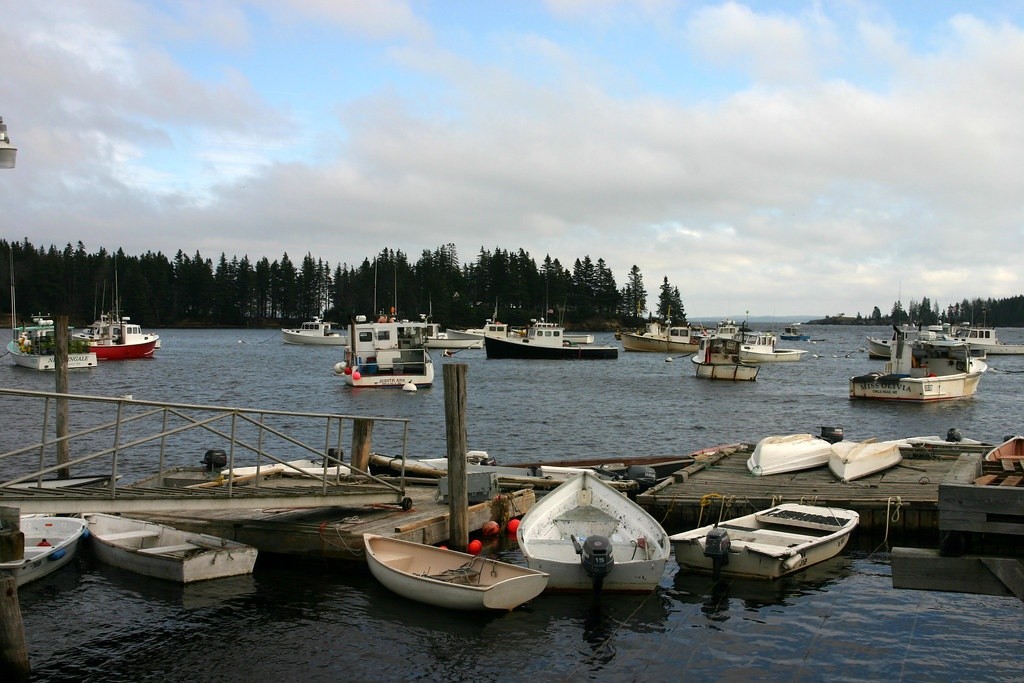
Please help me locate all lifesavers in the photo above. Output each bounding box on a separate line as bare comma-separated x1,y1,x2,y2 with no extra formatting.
347,352,350,366
733,356,739,362
563,340,571,345
691,339,697,344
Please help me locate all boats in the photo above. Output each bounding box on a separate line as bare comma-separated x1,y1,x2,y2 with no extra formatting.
848,323,987,403
781,323,810,341
984,434,1024,461
363,531,550,613
614,298,718,352
73,310,159,359
514,471,671,594
691,336,759,382
881,428,980,452
483,317,618,360
828,437,902,482
421,318,486,349
732,321,807,363
509,321,596,345
668,502,859,583
82,511,258,585
335,307,434,388
906,432,998,451
864,324,1024,359
0,515,87,585
281,314,347,345
744,429,832,478
5,312,97,372
